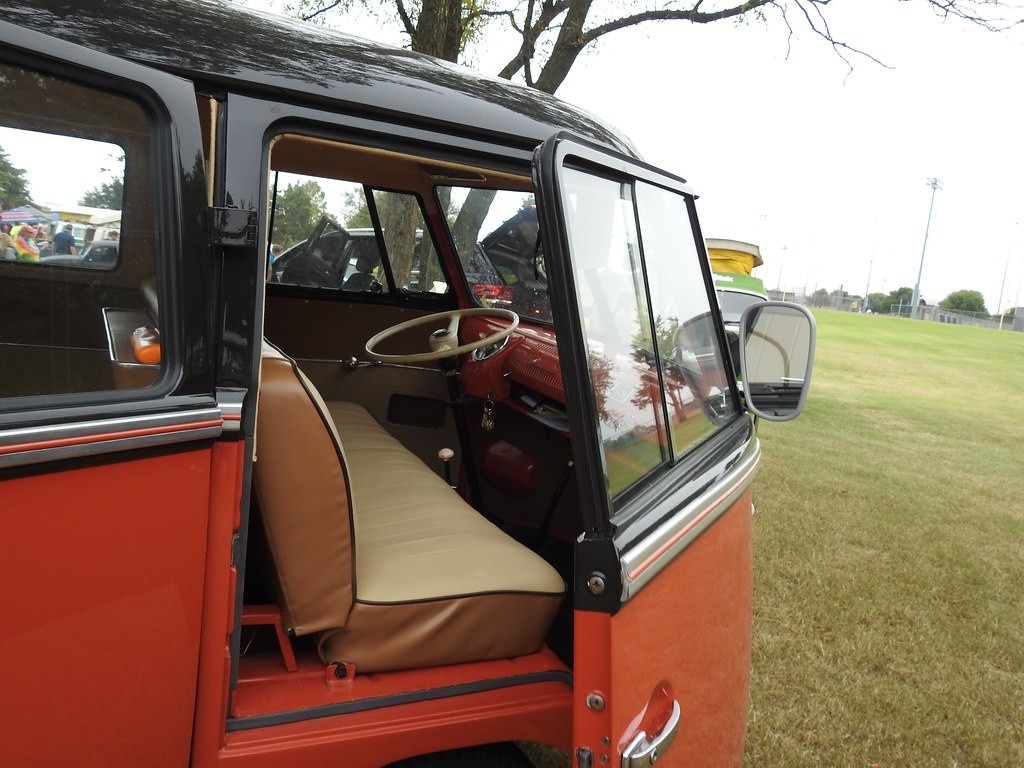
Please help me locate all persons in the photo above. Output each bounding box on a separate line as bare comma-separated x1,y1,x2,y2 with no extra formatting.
102,230,118,242
0,222,77,262
267,244,284,282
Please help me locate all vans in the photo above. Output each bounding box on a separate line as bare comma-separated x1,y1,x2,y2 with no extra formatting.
0,1,818,768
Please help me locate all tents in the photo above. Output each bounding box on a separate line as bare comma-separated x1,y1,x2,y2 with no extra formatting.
0,205,56,226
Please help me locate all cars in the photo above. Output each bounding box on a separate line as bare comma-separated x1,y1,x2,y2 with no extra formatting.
38,240,118,270
267,204,555,325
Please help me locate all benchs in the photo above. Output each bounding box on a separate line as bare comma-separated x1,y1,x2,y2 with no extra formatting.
253,335,566,676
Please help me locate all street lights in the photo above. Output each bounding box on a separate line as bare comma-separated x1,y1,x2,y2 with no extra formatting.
908,176,943,320
